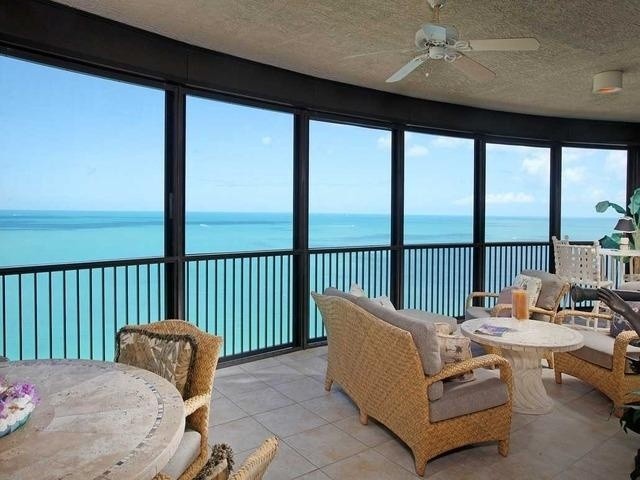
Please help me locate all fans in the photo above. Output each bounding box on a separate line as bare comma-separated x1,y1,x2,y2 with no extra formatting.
340,0,541,83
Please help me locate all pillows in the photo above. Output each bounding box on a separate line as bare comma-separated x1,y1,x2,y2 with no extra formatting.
497,287,526,318
610,301,640,346
371,294,397,312
348,284,368,299
514,274,542,318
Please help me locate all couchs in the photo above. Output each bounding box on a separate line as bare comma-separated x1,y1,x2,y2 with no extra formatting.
554,282,640,417
396,309,458,335
311,287,514,477
466,269,570,369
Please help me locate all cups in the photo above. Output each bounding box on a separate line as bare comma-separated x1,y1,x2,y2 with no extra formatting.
511,290,528,319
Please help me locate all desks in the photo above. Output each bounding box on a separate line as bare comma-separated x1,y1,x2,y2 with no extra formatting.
0,358,185,480
599,250,640,289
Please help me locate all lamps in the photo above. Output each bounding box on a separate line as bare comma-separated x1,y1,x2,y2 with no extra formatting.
614,216,637,245
592,70,623,94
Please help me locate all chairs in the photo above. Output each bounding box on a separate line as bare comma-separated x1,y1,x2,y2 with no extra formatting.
192,443,233,480
114,319,224,480
228,435,280,480
553,238,614,330
